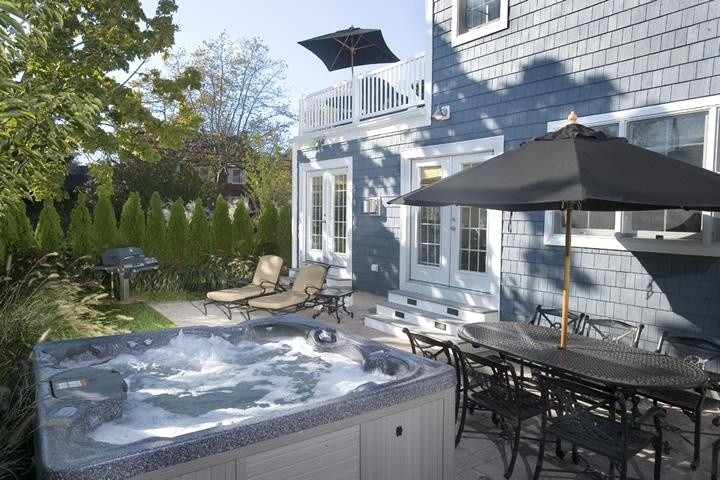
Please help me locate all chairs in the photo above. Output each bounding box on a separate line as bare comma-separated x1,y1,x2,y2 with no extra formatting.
403,327,452,365
450,345,565,479
656,330,719,426
579,315,644,348
247,262,330,319
204,254,283,320
526,304,588,335
531,368,666,480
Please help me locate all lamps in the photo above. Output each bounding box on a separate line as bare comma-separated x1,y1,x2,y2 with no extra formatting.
432,104,447,120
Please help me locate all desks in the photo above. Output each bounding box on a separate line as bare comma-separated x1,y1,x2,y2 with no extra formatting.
312,288,354,323
458,320,706,388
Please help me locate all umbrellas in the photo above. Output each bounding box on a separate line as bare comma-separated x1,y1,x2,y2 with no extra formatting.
386,110,720,417
296,25,400,78
